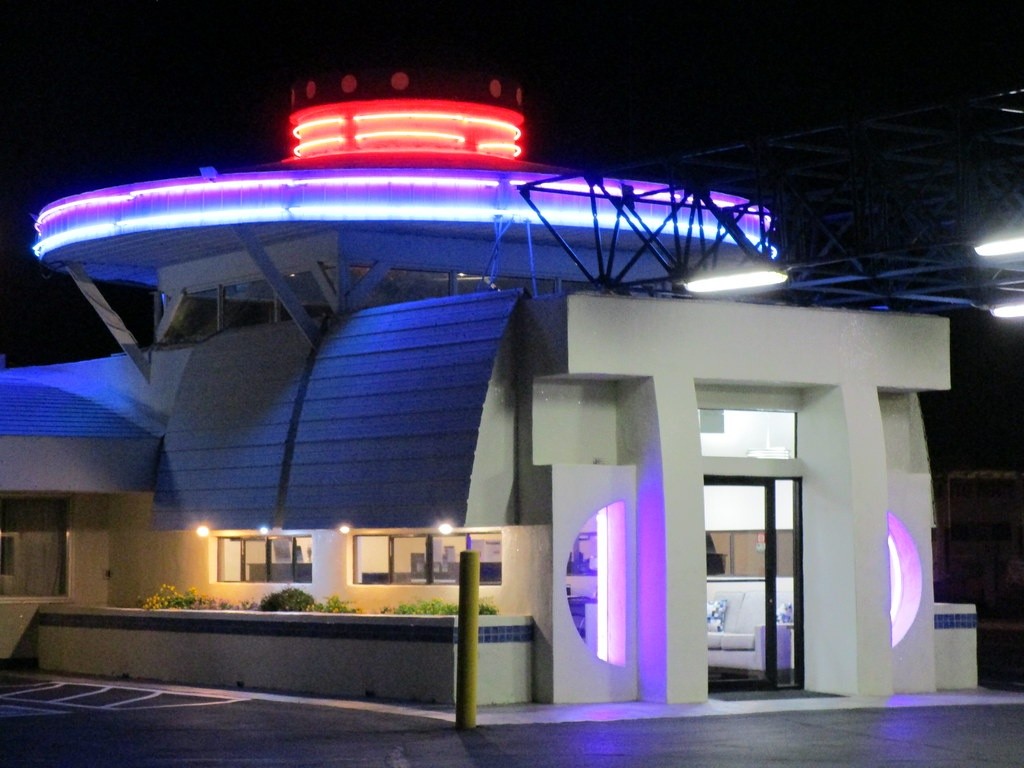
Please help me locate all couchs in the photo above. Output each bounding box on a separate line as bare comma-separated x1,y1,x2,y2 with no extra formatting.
703,589,797,685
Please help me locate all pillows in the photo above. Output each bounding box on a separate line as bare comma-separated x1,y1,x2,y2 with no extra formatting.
706,599,727,634
